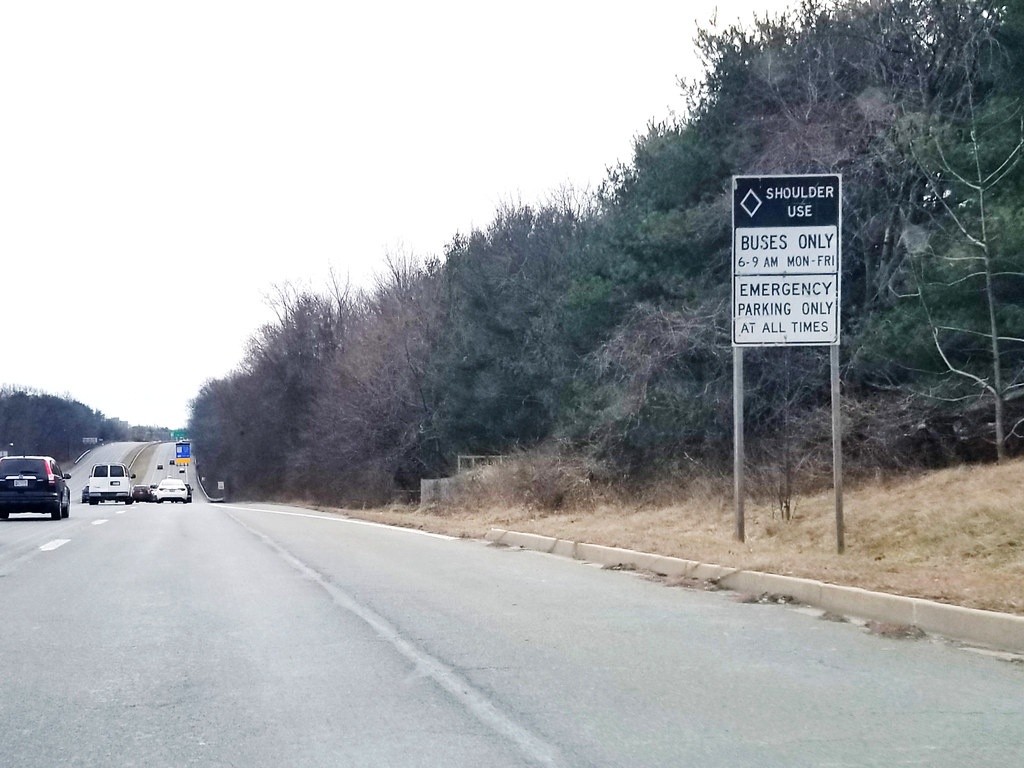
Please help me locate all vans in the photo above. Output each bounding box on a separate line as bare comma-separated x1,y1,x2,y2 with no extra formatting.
89,462,136,505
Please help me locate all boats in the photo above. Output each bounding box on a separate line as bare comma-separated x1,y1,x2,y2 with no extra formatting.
175,484,194,503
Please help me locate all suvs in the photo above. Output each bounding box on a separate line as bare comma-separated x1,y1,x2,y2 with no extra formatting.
0,456,71,521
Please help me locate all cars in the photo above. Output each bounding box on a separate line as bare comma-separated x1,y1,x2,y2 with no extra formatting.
82,486,89,503
149,485,159,503
132,485,151,503
157,479,187,503
